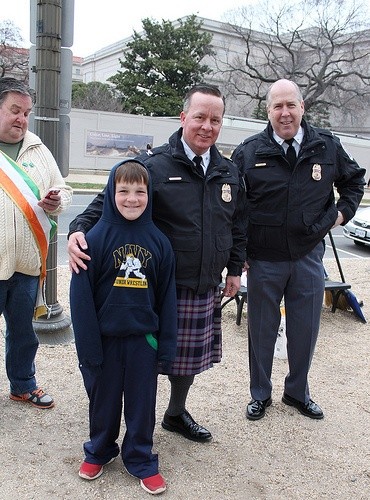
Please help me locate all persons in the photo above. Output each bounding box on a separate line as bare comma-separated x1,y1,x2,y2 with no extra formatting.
1,76,72,409
70,160,178,494
229,78,366,422
65,84,248,443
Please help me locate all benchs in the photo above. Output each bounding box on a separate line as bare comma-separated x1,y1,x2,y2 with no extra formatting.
324,279,354,313
219,282,248,326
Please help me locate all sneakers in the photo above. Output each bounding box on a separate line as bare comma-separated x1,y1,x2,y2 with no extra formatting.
9,387,55,409
140,473,166,495
78,457,117,481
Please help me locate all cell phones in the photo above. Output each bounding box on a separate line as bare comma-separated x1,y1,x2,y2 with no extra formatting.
43,188,59,201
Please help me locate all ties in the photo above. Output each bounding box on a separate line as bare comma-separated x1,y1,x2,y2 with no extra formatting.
193,156,205,178
284,138,297,167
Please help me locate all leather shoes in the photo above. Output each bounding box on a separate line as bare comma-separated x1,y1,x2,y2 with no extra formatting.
161,409,213,441
246,395,272,420
282,390,324,419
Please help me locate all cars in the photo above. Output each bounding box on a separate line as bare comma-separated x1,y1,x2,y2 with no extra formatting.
341,206,370,248
